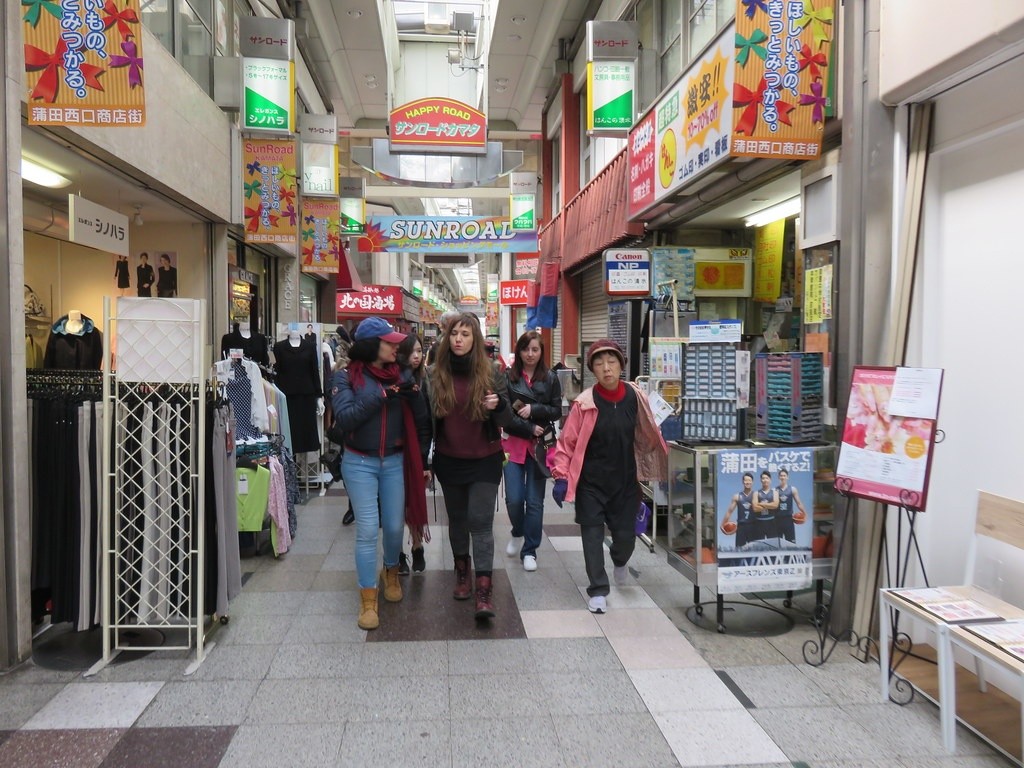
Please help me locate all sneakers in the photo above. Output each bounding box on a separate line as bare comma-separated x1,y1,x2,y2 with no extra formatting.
398,552,411,575
523,555,537,572
411,546,425,573
358,588,379,630
614,564,629,585
506,536,523,555
588,596,606,614
380,564,403,601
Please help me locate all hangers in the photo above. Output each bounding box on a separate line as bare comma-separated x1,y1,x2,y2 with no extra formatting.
26,366,229,410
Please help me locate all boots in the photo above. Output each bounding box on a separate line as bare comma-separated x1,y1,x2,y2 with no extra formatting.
453,555,473,599
475,576,495,618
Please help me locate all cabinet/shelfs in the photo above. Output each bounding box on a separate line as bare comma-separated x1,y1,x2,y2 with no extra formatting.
754,351,826,444
663,438,843,624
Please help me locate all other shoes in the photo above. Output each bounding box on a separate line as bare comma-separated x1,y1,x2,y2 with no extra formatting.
342,510,355,526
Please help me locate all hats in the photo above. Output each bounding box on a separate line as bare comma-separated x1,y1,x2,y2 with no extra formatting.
587,339,625,372
354,316,408,344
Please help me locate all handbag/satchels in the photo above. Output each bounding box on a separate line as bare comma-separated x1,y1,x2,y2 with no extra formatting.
319,449,342,482
634,502,650,536
536,425,557,463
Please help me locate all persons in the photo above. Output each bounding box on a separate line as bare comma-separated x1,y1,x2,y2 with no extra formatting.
501,331,563,571
305,324,317,351
137,253,155,298
156,254,178,298
720,469,754,548
774,469,808,544
550,338,669,614
752,471,779,540
275,330,324,454
428,315,507,621
399,311,481,471
222,322,274,384
113,255,131,296
330,317,432,630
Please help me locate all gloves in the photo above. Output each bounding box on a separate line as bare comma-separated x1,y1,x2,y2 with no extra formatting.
552,479,568,508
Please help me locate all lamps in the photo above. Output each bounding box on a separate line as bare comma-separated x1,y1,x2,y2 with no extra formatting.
22,154,74,189
130,204,145,226
742,193,801,228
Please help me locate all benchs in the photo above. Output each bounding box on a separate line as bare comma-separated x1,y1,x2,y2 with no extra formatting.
871,488,1024,768
